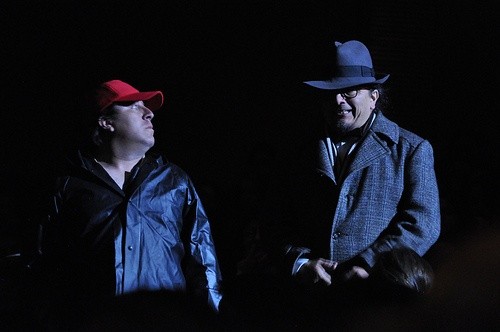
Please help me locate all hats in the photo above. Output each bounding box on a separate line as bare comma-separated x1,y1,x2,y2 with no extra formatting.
301,40,390,90
82,79,164,119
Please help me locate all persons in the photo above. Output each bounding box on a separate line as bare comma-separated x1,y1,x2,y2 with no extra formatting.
269,40,441,296
35,78,224,314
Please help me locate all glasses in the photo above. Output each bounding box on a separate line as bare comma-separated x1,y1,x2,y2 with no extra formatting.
342,87,358,99
